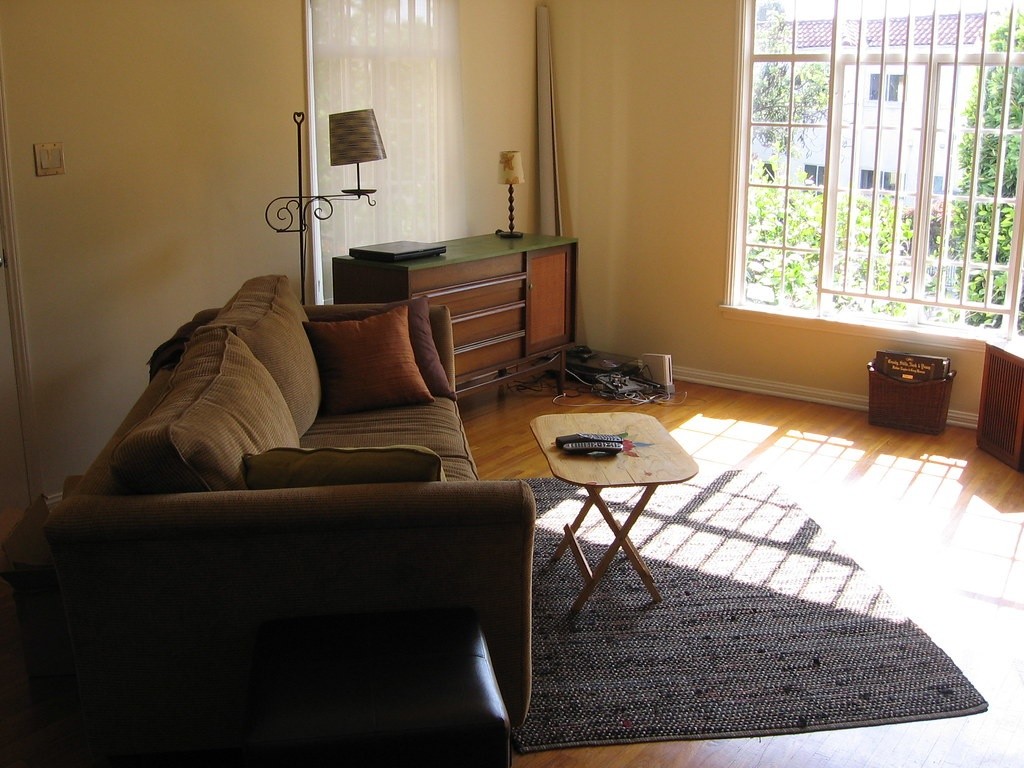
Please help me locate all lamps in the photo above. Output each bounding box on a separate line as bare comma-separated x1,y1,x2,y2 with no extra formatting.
494,151,525,238
265,109,387,305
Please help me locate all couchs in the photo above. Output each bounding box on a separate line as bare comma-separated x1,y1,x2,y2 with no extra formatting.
38,304,536,759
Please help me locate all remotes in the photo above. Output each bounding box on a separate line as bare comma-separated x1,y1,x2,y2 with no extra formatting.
563,442,624,453
556,434,623,447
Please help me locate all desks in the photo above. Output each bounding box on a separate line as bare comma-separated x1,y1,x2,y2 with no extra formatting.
977,343,1024,471
529,412,699,616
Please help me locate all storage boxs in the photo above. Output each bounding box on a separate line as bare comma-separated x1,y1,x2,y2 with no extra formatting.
0,474,84,707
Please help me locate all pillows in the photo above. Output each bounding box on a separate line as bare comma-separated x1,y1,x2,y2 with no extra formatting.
307,296,458,402
302,304,436,416
241,443,447,491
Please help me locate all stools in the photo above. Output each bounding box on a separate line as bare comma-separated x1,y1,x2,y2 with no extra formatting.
247,606,513,768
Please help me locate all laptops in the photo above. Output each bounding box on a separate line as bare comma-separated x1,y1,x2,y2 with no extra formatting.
349,242,447,263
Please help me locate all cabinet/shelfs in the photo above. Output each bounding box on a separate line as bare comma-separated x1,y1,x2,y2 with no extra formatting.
332,231,578,397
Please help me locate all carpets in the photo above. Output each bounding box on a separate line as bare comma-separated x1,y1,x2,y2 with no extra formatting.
519,469,989,753
